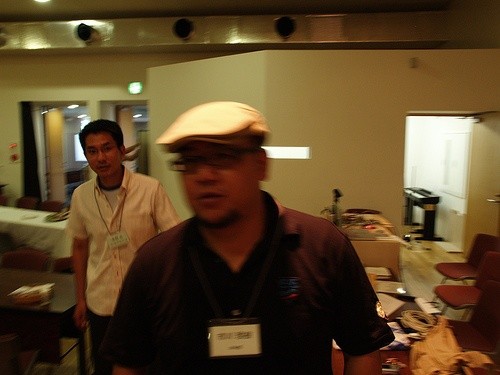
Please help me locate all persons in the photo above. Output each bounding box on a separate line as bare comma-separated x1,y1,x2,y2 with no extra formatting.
99,101,396,375
66,119,182,375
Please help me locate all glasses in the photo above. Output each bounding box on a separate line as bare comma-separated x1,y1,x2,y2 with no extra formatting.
168,147,260,172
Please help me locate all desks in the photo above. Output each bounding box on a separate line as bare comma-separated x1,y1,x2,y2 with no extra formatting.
335,211,406,276
333,262,474,375
0,206,75,272
0,264,88,375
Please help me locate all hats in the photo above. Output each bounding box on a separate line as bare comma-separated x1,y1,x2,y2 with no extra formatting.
154,101,271,149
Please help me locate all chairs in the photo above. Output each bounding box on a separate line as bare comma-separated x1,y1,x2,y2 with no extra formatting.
430,233,500,375
0,191,74,375
